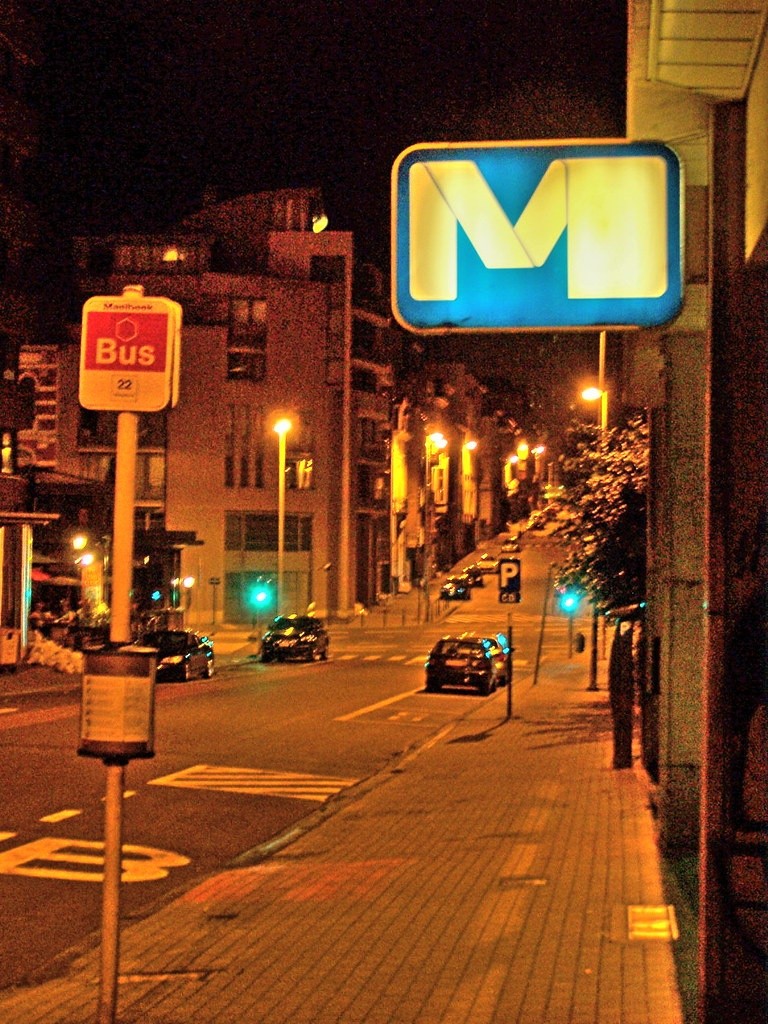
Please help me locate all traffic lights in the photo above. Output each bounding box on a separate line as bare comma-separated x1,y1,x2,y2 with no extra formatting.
252,579,267,606
562,585,573,610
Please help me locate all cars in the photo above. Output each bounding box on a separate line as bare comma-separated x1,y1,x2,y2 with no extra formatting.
261,616,332,662
439,499,557,599
425,637,497,695
461,632,515,686
137,631,215,681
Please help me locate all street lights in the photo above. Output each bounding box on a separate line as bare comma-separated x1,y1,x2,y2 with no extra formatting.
582,388,609,438
273,419,291,615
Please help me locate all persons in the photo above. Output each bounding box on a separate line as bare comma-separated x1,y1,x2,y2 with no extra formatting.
29,595,110,638
